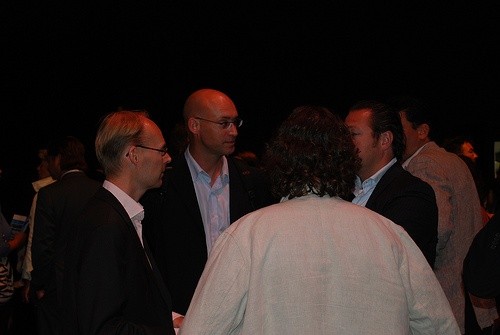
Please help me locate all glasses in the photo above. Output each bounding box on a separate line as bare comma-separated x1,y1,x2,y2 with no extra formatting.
194,117,243,128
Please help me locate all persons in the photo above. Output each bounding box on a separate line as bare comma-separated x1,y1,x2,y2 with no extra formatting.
176,104,461,335
0,88,277,335
343,101,500,335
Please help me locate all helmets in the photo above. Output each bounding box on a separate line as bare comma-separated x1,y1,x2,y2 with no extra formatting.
126,145,168,157
